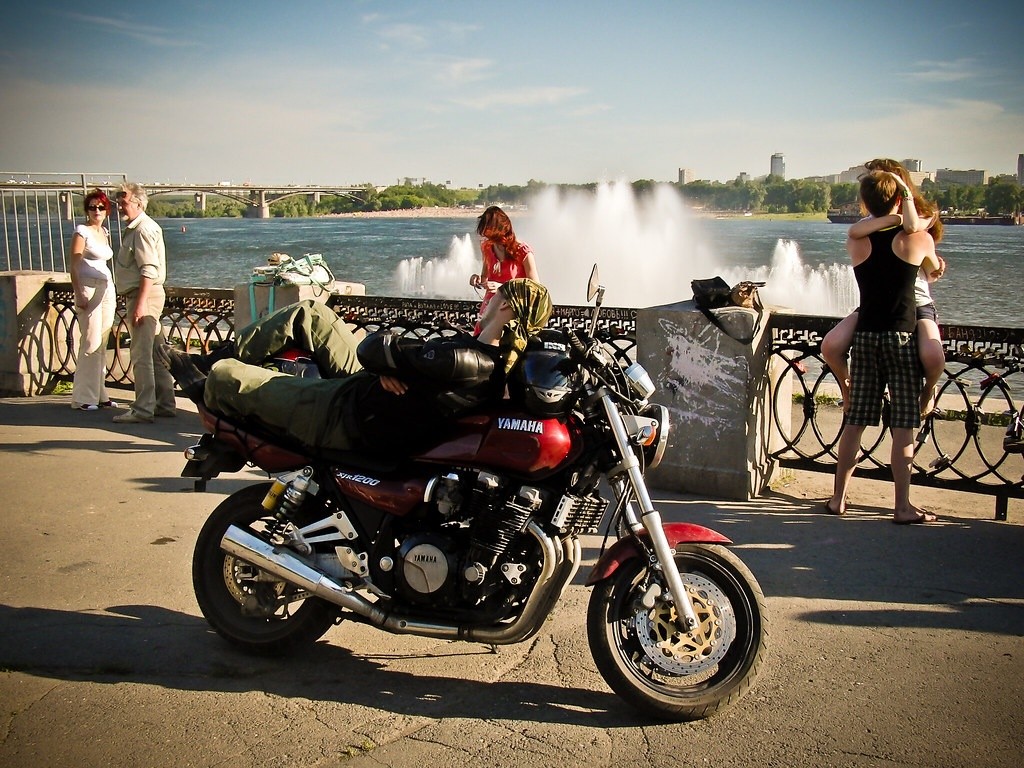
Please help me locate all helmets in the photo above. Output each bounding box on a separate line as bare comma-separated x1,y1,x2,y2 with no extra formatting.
506,329,580,418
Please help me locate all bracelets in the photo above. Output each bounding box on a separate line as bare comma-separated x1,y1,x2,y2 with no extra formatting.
902,189,915,201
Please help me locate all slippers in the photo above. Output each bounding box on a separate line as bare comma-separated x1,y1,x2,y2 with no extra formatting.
824,500,846,515
893,509,938,526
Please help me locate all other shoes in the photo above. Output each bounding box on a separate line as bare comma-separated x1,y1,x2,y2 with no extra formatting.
113,410,154,423
153,408,176,417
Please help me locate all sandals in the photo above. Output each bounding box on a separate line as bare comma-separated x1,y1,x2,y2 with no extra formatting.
97,400,118,409
71,401,98,411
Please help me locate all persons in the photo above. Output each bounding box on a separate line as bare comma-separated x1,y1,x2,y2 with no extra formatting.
159,278,552,460
110,182,177,422
70,190,116,409
473,206,541,339
820,159,946,524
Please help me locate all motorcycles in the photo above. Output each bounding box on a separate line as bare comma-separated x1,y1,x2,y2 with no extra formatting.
158,263,769,720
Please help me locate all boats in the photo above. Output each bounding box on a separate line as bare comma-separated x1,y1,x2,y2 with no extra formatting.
827,203,1024,226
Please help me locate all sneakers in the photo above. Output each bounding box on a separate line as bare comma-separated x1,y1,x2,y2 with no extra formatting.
205,340,235,366
155,344,208,405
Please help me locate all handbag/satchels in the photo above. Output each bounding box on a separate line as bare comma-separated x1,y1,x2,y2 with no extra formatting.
730,281,767,308
690,276,763,345
250,266,278,324
273,254,336,292
267,253,289,267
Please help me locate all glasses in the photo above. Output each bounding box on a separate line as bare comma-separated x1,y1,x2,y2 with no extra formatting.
87,205,107,211
474,275,493,300
115,191,142,203
